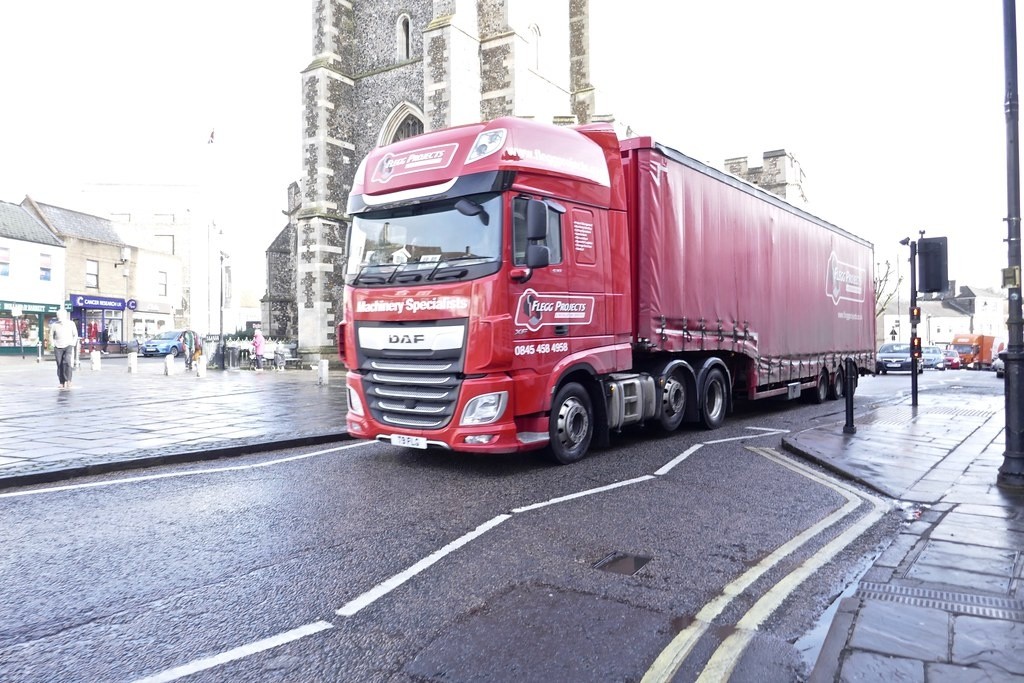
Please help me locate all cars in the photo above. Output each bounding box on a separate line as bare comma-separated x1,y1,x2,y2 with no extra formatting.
922,347,945,370
141,330,197,357
876,343,923,375
996,358,1005,378
944,350,960,369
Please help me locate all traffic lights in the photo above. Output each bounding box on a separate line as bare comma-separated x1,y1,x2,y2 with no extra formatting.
911,337,922,358
909,307,921,324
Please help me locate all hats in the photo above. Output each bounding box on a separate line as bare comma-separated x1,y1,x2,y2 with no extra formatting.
253,330,262,336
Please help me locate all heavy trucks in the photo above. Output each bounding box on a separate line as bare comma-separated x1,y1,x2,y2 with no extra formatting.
338,118,876,466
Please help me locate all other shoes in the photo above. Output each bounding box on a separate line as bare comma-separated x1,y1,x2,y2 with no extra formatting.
59,382,72,388
185,364,193,369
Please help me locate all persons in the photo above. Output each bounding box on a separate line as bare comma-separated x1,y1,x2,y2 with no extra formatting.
48,309,78,388
250,328,265,372
177,328,205,372
100,327,109,355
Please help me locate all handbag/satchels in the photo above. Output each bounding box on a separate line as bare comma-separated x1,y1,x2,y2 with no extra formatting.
193,350,201,361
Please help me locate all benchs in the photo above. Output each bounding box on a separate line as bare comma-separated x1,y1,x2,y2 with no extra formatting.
118,339,143,354
249,345,304,371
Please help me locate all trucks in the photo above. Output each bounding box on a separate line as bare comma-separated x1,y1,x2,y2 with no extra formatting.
949,334,995,370
